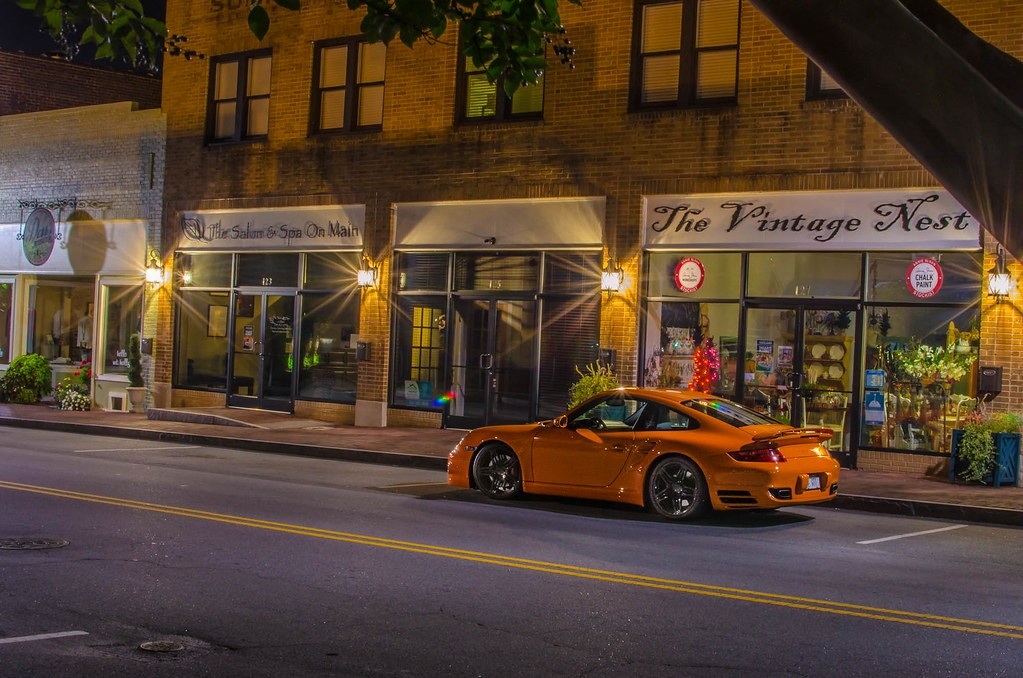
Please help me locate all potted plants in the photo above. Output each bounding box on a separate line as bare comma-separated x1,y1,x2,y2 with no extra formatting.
949,413,1023,488
2,353,54,403
127,334,148,413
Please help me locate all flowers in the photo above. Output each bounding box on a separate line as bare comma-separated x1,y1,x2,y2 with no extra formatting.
56,358,91,410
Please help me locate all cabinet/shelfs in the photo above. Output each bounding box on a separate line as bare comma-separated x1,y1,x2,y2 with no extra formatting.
784,332,853,450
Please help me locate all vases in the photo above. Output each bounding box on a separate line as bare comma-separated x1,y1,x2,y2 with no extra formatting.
82,390,91,406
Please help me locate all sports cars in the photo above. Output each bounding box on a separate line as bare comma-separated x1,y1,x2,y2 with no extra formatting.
447,386,841,523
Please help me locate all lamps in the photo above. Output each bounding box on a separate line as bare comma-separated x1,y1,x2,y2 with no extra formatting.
357,259,373,286
599,256,624,299
986,250,1011,303
146,249,161,283
